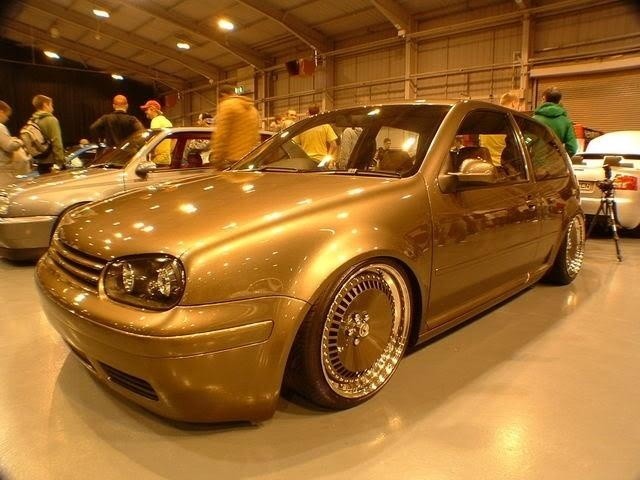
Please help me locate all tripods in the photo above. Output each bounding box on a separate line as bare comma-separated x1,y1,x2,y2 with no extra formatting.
586,193,625,262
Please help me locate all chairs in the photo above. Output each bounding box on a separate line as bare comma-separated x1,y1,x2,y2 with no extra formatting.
377,148,492,176
185,151,203,168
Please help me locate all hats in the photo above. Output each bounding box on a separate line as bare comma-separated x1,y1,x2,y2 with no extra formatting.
140,100,161,111
113,95,128,107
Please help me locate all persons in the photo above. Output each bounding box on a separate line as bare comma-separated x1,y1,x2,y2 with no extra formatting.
479,92,519,169
210,86,261,169
0,98,24,164
270,103,363,168
524,87,578,168
376,138,391,161
89,95,144,146
187,112,213,165
30,95,66,171
139,100,173,167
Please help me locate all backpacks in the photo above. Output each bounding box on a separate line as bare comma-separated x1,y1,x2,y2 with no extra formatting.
20,115,53,157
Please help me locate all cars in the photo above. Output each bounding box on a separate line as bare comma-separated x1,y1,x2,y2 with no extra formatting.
29,99,587,428
21,143,105,176
566,130,639,234
0,126,312,264
